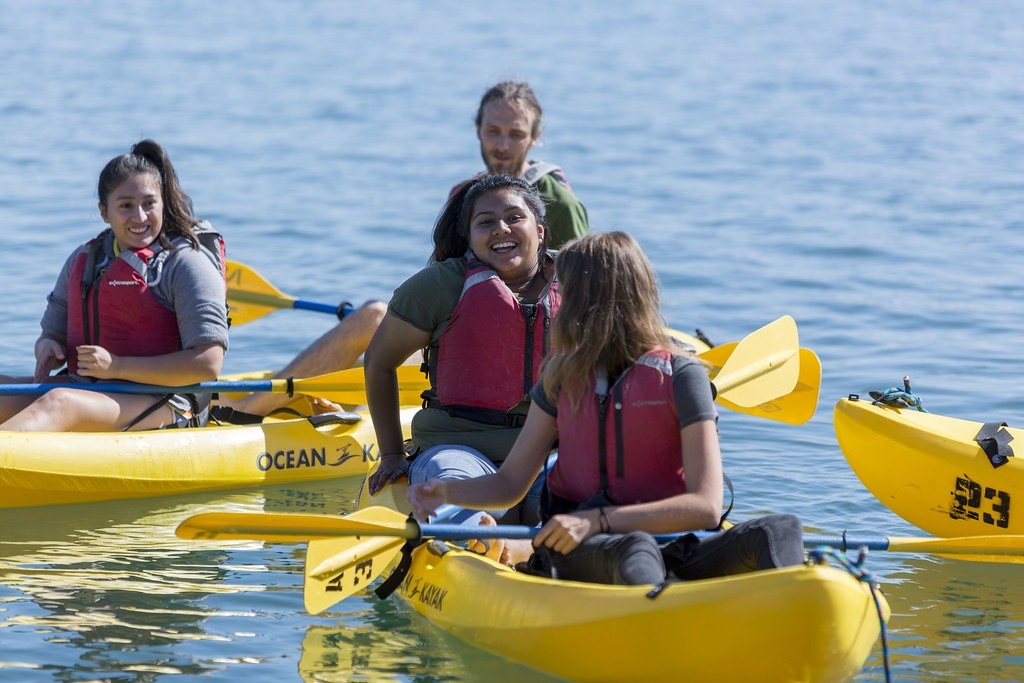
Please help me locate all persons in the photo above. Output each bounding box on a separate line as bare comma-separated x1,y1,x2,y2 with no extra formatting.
0,139,228,437
405,229,806,584
363,171,576,522
210,79,588,425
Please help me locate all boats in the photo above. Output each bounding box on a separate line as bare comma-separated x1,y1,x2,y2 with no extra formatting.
833,377,1024,538
0,325,892,683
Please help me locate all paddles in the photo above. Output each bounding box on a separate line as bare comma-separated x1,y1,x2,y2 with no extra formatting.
303,314,799,615
0,364,435,409
175,509,1024,564
226,257,821,425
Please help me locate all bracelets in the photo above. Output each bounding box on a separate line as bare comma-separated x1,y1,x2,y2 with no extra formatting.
599,506,611,534
